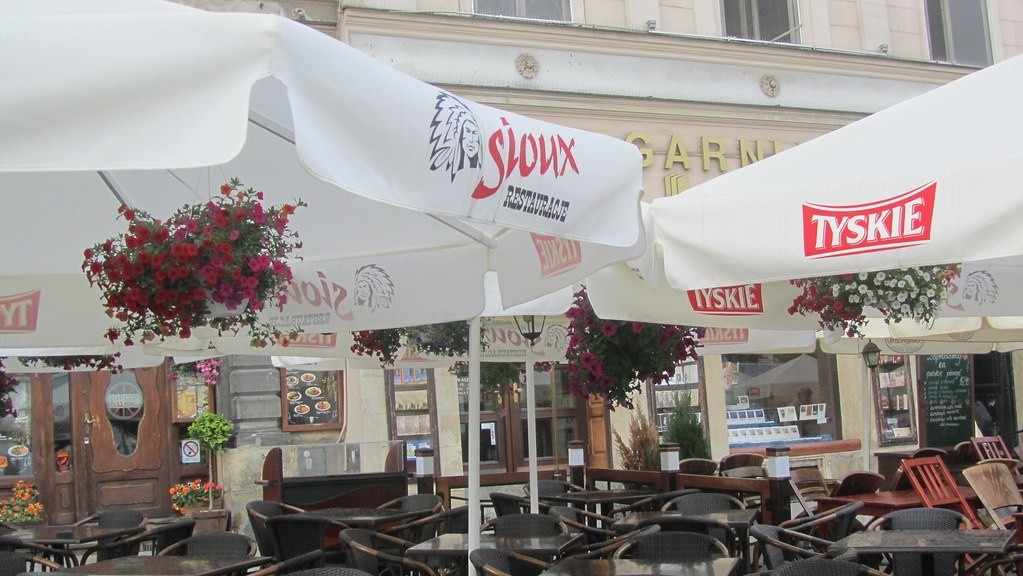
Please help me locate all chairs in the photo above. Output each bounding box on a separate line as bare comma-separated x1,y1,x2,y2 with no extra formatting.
0,436,1023,576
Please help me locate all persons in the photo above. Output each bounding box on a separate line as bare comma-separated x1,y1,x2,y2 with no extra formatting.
723,367,737,405
784,387,814,437
670,391,676,405
659,392,665,406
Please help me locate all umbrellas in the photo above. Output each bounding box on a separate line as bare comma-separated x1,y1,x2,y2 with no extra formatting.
576,54,1022,355
732,353,819,401
0,0,647,576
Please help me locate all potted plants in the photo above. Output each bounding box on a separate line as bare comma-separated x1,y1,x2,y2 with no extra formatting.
187,412,235,534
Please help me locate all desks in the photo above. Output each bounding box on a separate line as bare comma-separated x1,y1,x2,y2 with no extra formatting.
53,555,273,576
288,507,435,534
538,489,662,529
404,534,588,576
873,445,956,491
0,526,265,568
538,557,742,576
812,484,983,528
611,509,761,576
827,530,1016,576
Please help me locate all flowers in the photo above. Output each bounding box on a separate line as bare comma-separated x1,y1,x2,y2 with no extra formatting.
170,479,222,514
0,176,305,420
563,282,707,411
350,318,490,368
788,262,959,340
448,361,554,418
0,480,48,522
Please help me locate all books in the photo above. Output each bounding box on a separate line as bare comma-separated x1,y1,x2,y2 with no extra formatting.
891,393,908,411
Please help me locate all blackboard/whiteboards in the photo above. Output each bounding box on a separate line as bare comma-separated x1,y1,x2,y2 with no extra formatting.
920,353,975,446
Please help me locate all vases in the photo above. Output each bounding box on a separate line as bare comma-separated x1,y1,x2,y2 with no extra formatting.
201,280,249,319
418,333,432,345
185,499,222,517
9,520,44,553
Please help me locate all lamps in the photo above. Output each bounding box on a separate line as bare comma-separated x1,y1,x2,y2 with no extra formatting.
860,339,882,372
513,315,547,347
